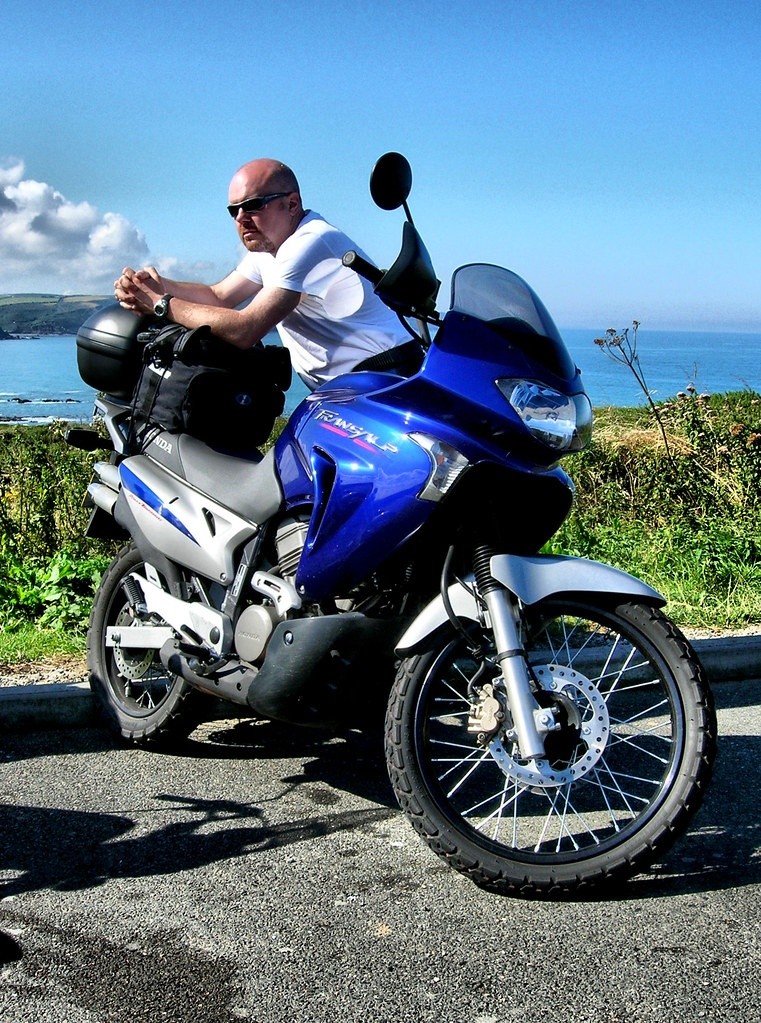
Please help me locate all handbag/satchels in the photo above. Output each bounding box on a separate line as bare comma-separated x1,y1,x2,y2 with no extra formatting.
131,322,292,447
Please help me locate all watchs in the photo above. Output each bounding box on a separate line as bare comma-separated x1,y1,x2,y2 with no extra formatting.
151,293,174,326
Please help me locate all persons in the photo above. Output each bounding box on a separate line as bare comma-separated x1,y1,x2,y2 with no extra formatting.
114,159,428,395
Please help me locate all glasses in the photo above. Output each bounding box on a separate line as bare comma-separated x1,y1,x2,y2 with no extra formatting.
227,191,294,218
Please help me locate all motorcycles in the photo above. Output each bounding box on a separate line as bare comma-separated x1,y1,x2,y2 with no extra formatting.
67,152,721,901
